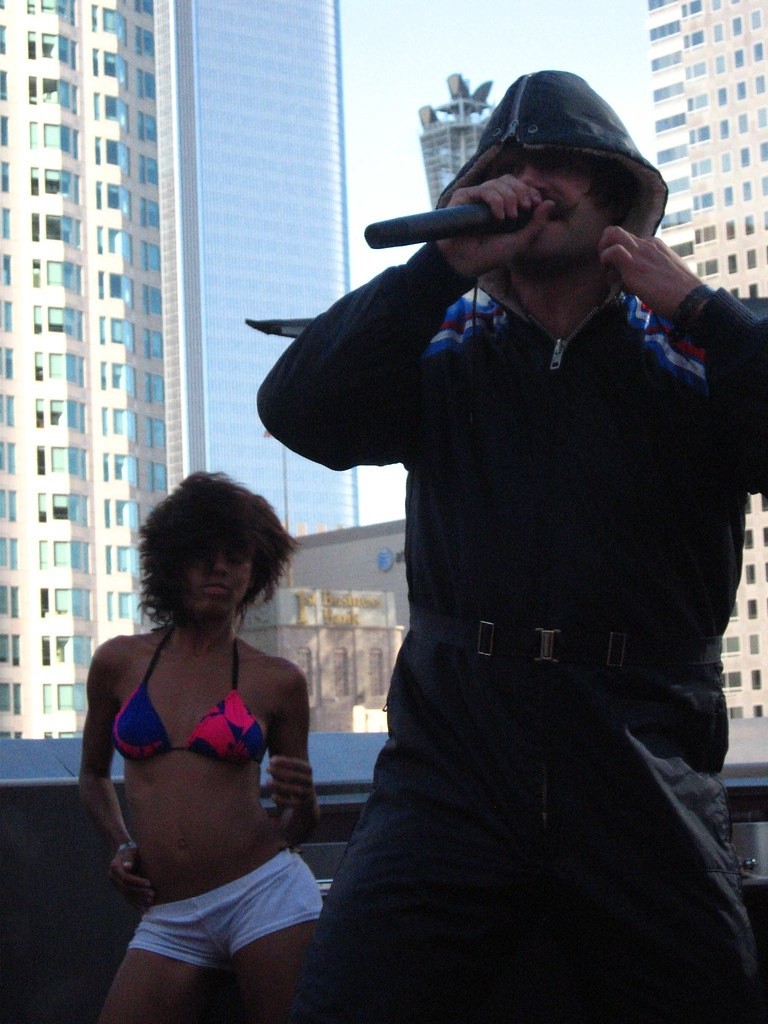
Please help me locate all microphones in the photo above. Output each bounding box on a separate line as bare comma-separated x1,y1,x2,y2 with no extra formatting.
365,200,532,249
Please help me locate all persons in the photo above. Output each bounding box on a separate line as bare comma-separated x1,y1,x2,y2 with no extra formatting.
254,70,768,1024
77,470,323,1024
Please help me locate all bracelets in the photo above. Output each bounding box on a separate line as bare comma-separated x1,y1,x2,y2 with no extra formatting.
672,285,716,328
118,840,137,850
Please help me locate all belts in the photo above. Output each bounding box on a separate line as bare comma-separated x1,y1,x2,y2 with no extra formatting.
409,602,720,667
